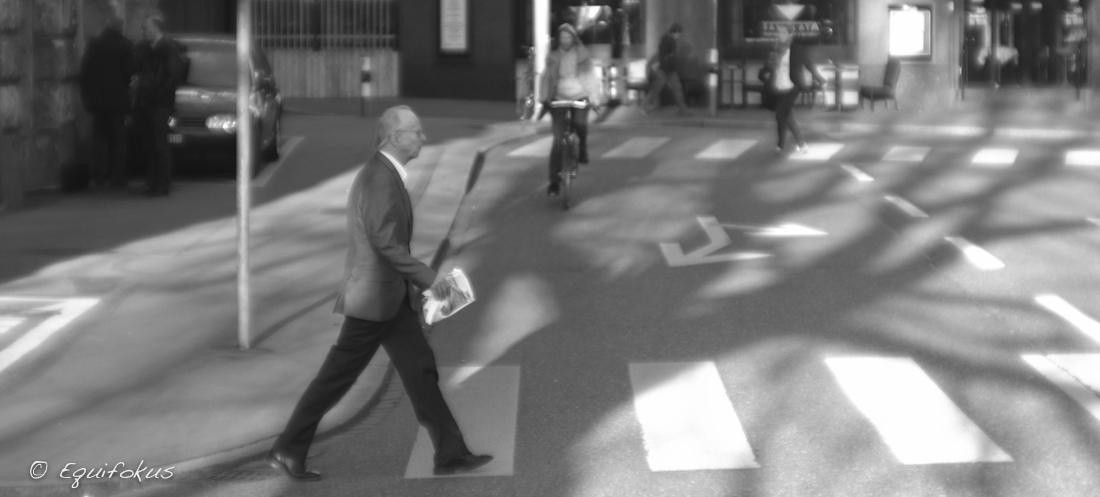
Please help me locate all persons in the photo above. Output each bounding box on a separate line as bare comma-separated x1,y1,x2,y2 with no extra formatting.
758,25,825,156
536,23,601,196
265,105,495,481
80,17,135,193
127,16,190,197
640,22,694,117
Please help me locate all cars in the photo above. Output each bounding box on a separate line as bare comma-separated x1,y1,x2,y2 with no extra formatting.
164,34,284,177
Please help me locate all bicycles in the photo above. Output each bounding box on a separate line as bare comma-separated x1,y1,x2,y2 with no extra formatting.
522,96,606,207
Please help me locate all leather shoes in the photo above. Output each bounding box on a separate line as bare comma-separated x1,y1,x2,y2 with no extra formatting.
265,446,321,479
434,452,493,474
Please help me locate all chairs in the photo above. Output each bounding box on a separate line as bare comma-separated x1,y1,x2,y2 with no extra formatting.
626,53,903,111
